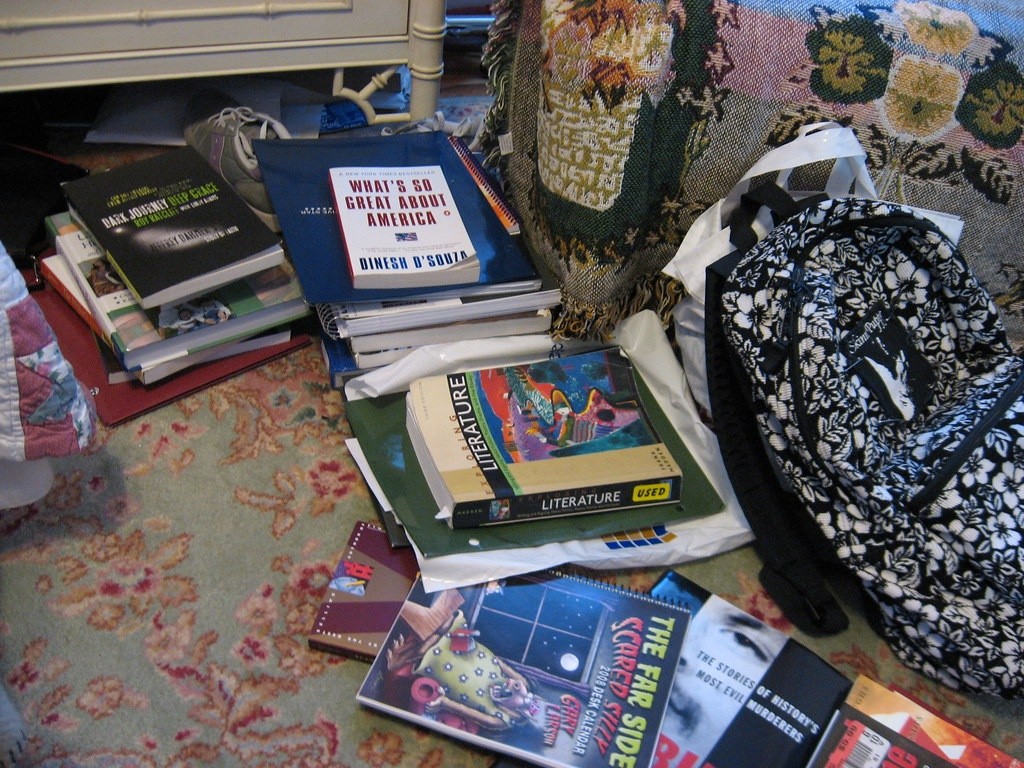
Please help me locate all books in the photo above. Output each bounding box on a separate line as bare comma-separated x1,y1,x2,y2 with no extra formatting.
42,131,1023,768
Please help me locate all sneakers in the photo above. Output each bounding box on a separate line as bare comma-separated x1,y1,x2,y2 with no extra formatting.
182,88,291,233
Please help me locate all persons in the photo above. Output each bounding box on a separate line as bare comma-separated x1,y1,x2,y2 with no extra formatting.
649,594,790,768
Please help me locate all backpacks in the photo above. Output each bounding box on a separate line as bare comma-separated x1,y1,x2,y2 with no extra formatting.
704,183,1024,698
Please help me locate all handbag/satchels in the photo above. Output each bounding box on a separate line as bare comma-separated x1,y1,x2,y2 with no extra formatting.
659,121,965,419
